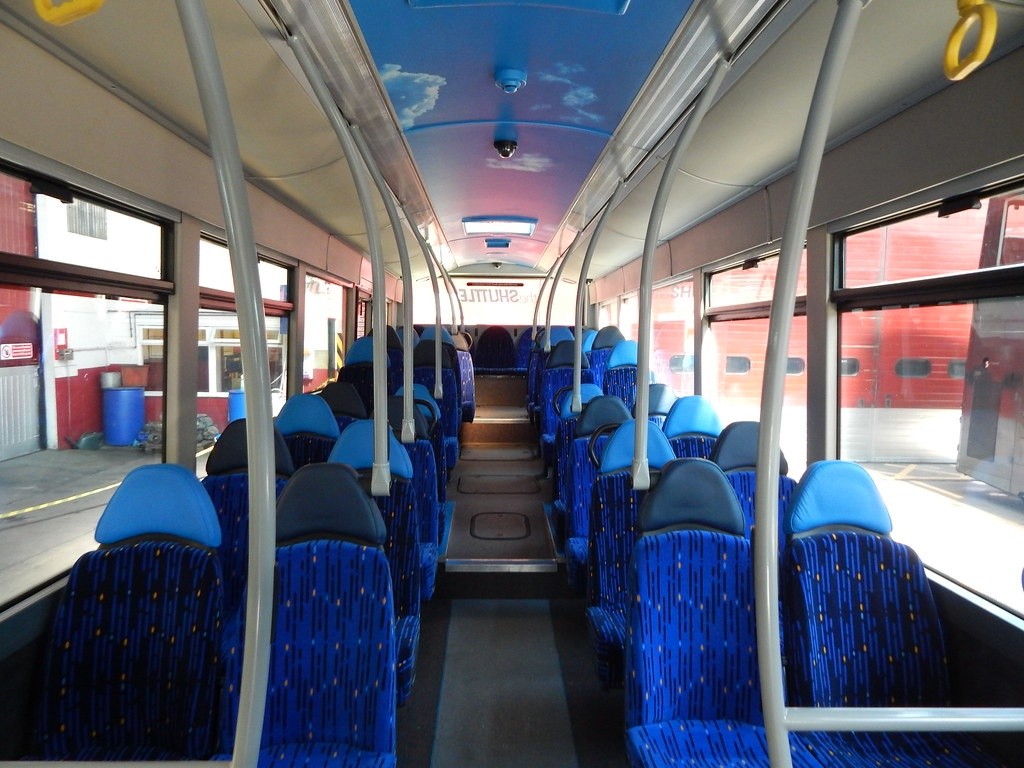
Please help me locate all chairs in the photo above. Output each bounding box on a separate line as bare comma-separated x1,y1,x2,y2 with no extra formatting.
36,322,1024,768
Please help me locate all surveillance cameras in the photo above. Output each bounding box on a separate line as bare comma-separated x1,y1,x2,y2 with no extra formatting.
493,262,501,268
493,129,519,158
